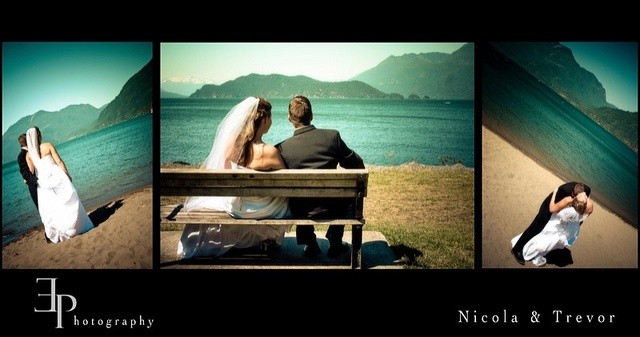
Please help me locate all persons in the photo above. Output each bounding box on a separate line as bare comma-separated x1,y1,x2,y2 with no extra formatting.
511,181,591,265
17,134,51,243
26,125,94,244
177,96,294,259
523,186,593,267
274,96,365,260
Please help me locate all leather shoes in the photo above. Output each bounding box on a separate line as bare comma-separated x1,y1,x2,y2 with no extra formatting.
327,240,350,258
511,247,526,264
303,244,322,256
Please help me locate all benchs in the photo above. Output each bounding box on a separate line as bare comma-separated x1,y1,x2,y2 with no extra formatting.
158,170,369,269
